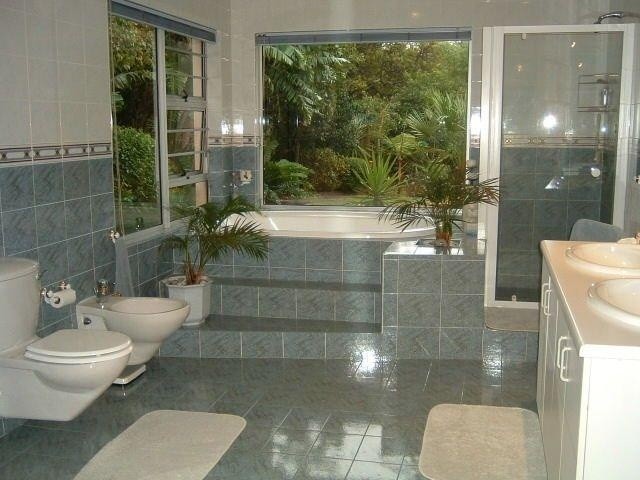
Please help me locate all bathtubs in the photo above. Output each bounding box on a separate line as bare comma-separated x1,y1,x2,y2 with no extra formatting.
219,208,441,240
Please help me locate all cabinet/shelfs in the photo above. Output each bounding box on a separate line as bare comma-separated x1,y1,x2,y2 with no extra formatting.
535,257,590,480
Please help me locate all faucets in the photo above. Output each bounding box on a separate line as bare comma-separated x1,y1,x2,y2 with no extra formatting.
95,277,121,301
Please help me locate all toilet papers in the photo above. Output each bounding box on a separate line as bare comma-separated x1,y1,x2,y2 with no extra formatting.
50,288,78,310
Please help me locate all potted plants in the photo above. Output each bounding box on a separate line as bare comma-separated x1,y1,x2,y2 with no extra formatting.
157,193,276,329
378,153,506,248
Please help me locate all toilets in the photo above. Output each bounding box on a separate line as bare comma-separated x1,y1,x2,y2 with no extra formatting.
0,254,133,424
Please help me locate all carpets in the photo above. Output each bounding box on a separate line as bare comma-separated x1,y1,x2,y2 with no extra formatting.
417,400,549,480
72,407,248,480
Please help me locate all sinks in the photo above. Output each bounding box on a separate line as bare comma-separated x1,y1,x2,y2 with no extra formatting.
565,240,636,277
78,293,192,367
586,277,636,331
